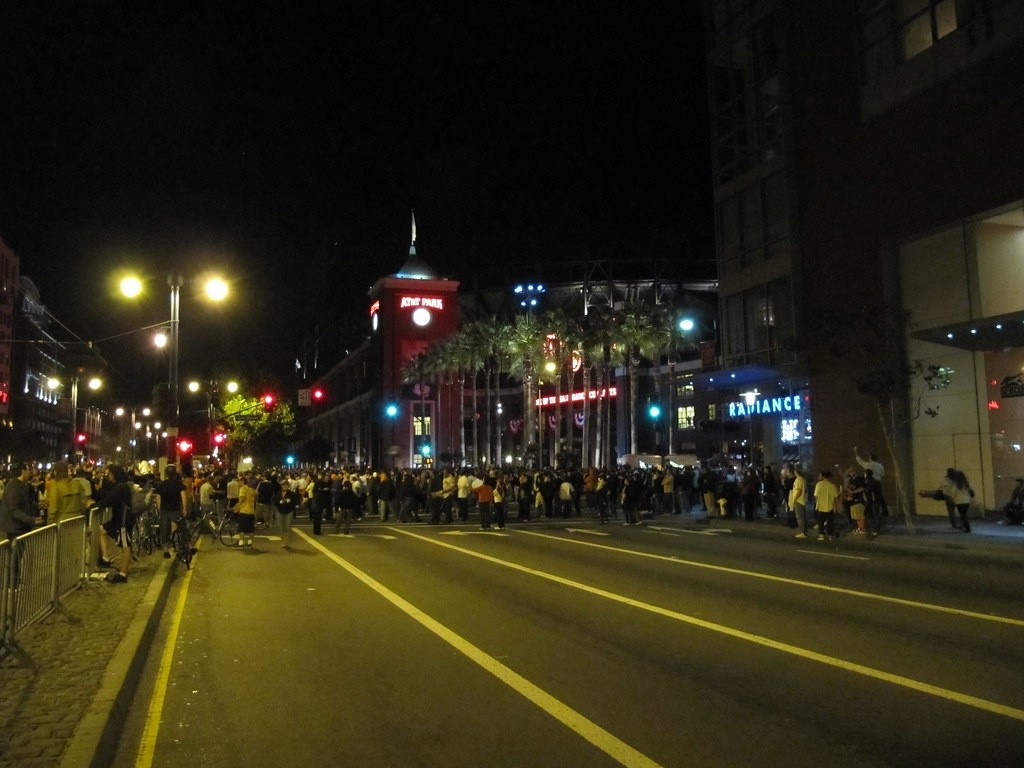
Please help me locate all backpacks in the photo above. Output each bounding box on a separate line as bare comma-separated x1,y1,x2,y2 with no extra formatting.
121,484,147,514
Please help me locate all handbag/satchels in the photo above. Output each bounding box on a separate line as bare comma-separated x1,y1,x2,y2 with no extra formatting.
933,486,945,501
968,489,975,498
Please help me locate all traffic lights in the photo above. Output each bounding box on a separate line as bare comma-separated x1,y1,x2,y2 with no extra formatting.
314,389,322,408
177,441,191,455
212,433,226,444
264,394,274,413
78,434,87,444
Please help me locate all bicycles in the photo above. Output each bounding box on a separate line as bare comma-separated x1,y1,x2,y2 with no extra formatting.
189,507,245,547
129,500,162,563
172,514,192,571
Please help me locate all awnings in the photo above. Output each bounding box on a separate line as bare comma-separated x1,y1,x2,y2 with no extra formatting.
688,364,786,393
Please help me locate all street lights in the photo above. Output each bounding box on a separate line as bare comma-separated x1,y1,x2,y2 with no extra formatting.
538,363,556,470
48,376,102,468
120,274,236,466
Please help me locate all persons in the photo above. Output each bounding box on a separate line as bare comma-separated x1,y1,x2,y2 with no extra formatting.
1010,479,1024,514
931,468,974,532
153,466,198,558
90,464,134,583
273,480,298,548
814,472,842,540
0,463,45,588
0,451,890,537
788,467,808,539
233,477,260,551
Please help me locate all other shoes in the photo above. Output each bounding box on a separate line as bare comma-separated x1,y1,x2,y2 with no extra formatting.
244,543,252,549
190,548,197,553
795,533,808,538
233,541,243,549
479,528,485,530
109,574,128,583
97,558,111,568
495,526,504,530
164,552,172,558
487,528,490,530
